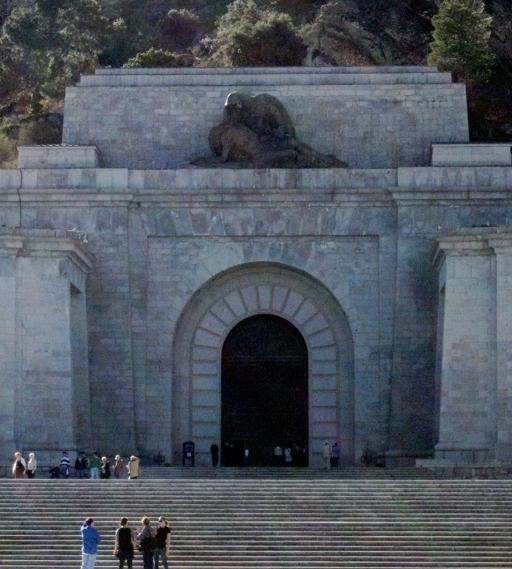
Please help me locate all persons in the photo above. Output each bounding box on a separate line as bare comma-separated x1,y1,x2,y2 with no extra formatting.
134,518,158,569
189,120,333,168
11,449,141,480
209,438,340,472
153,515,172,569
80,518,101,569
113,517,136,569
221,88,351,168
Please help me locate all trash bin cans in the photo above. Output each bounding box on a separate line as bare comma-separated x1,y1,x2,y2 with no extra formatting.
182,441,194,467
376,454,385,467
